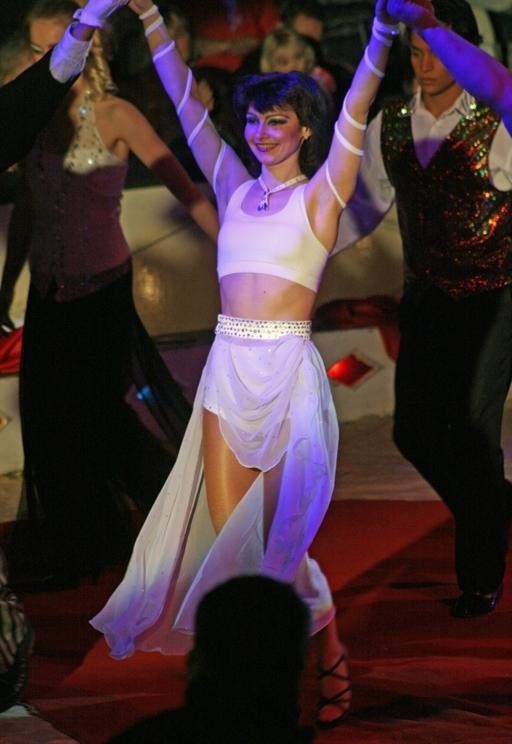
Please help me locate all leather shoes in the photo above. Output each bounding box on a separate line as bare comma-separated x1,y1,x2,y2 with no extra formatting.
451,587,502,618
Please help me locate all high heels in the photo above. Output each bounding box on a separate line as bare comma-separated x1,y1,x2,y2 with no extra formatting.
315,655,352,729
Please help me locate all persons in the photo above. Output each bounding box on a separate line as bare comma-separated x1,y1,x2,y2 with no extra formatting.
1,1,219,579
100,1,352,174
110,575,318,743
334,1,511,619
89,1,399,730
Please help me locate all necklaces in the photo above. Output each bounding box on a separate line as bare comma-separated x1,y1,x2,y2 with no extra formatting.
257,174,308,211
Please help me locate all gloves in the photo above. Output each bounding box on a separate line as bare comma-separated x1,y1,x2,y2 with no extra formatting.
72,0,129,28
